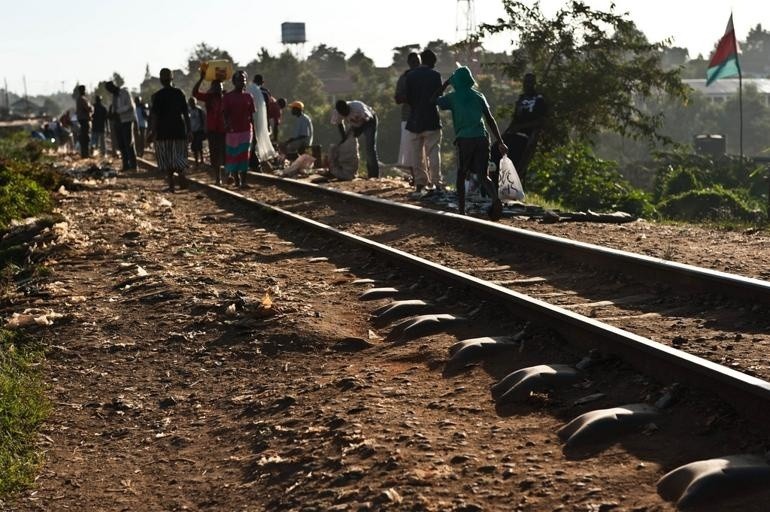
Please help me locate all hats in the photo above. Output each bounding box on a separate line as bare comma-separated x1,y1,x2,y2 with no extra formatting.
288,101,304,111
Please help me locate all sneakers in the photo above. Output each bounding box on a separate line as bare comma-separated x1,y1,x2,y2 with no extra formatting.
491,199,503,222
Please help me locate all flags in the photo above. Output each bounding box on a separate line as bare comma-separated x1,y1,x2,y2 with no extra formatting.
705,15,739,87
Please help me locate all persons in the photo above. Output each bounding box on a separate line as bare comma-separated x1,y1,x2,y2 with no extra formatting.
329,99,380,180
433,68,510,221
31,65,315,185
489,72,548,187
394,49,447,191
